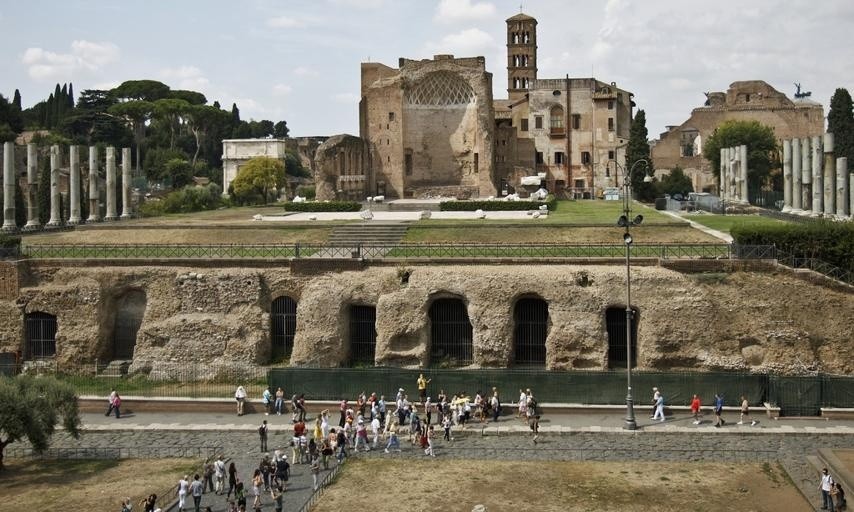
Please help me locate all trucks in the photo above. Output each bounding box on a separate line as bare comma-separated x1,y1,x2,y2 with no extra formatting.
751,198,765,205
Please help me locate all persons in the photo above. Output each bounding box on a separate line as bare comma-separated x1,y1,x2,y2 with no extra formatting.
119,496,132,511
818,468,835,512
690,394,701,425
104,387,121,415
713,393,725,428
346,372,541,458
737,394,756,426
111,391,121,418
828,483,846,512
650,391,666,422
137,384,346,511
651,387,660,418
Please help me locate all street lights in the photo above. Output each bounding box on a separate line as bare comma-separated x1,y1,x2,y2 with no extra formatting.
603,159,652,430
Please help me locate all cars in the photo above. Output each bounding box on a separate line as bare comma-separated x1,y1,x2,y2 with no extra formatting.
663,191,747,215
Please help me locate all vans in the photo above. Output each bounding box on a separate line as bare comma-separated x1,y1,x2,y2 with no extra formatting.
774,200,784,208
